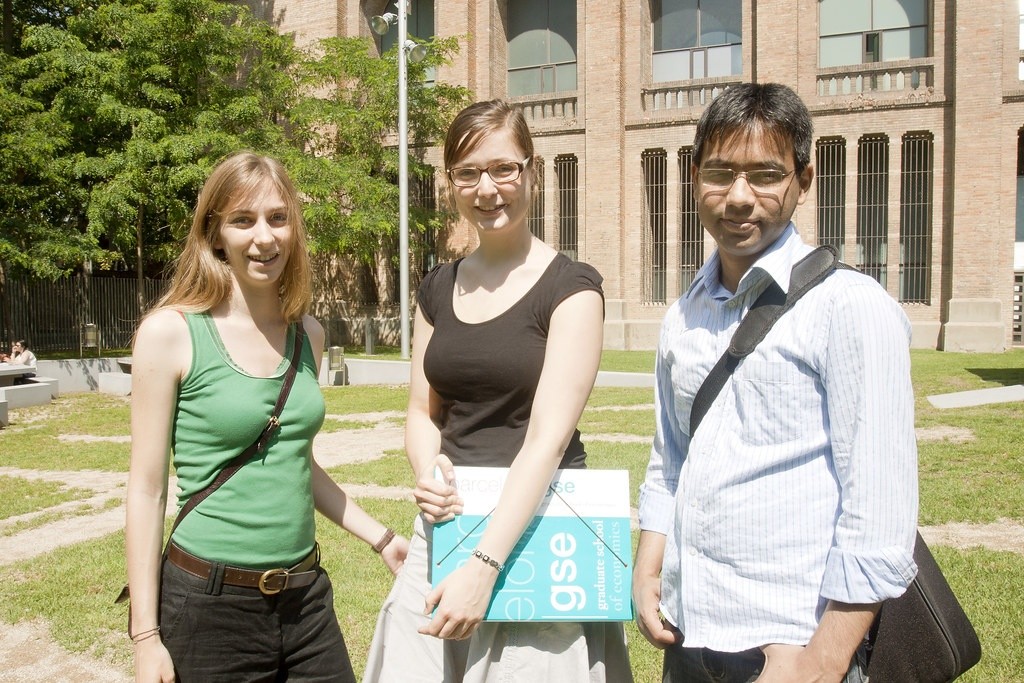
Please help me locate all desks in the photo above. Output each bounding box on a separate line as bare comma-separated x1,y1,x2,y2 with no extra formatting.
0,363,36,388
118,358,132,364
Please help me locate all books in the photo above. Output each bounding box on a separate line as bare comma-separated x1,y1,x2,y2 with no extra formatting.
430,468,634,621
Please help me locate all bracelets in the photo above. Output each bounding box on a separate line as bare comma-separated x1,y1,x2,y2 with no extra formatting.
472,549,505,572
372,529,395,554
131,626,160,645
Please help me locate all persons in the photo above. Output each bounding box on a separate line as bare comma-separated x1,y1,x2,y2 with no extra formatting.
126,150,411,683
632,82,918,682
3,340,37,382
357,99,632,683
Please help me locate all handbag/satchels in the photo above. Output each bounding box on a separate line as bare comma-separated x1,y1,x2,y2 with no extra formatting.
114,582,133,638
855,535,985,683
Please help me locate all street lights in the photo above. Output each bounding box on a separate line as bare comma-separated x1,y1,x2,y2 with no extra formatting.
371,0,428,358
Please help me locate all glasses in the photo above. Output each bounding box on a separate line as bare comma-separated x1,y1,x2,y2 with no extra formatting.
694,166,802,191
446,154,534,189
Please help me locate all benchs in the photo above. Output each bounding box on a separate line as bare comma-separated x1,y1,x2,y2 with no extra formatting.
0,377,59,425
99,373,131,396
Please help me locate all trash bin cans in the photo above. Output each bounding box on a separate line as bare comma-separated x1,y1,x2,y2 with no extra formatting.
327,345,345,386
83,323,97,348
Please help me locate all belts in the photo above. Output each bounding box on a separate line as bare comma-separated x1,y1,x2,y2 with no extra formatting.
168,540,321,595
